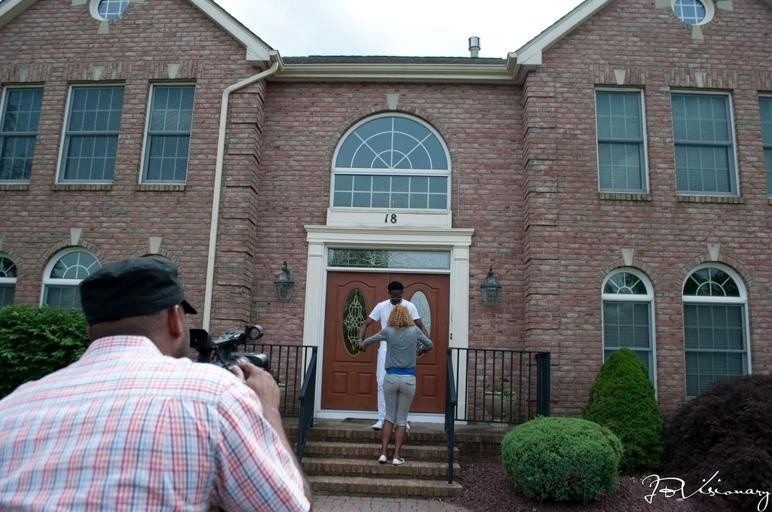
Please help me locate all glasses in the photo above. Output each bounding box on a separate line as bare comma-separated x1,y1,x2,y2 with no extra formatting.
390,295,402,300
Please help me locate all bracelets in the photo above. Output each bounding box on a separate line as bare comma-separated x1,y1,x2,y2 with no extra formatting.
418,344,423,346
419,344,424,350
358,335,365,340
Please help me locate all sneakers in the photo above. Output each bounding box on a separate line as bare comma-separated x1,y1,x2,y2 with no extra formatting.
378,455,387,463
372,420,384,430
392,458,405,465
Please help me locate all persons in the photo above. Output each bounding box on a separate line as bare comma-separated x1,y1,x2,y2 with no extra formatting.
358,302,434,466
356,280,434,432
0,253,315,512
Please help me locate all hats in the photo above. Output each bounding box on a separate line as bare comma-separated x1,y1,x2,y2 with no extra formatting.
78,254,198,327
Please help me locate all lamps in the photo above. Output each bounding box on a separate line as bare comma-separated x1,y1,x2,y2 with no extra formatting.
273,260,296,300
480,264,503,306
